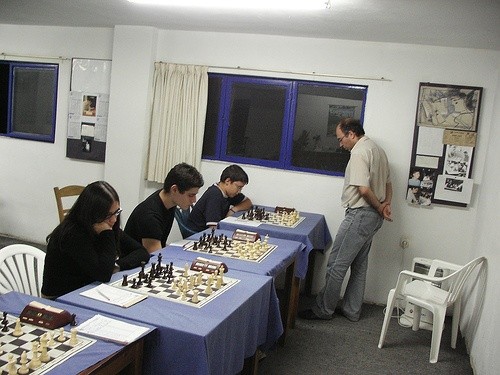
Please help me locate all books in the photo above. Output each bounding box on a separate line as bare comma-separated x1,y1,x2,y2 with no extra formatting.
72,313,149,346
79,283,149,308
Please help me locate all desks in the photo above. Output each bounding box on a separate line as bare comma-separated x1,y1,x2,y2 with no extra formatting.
0,291,158,375
55,257,274,375
151,228,307,349
219,204,332,329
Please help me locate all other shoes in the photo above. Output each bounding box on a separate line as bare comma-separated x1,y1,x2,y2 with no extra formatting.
297,308,332,320
335,307,358,321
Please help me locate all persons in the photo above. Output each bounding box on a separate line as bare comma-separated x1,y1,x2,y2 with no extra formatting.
124,162,204,253
296,116,393,323
40,181,151,301
184,163,253,238
406,171,433,206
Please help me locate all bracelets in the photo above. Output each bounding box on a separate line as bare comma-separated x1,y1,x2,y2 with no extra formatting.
230,208,236,215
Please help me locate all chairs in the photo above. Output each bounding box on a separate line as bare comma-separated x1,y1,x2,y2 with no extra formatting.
378,255,485,365
175,203,197,240
0,243,46,298
54,185,85,224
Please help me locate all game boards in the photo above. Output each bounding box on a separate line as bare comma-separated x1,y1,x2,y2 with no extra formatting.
110,264,242,309
183,236,278,263
236,209,307,230
0,311,98,375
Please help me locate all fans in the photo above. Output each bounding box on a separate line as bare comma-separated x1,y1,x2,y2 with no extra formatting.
400,256,449,332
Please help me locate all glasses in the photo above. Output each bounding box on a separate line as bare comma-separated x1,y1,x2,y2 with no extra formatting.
105,208,123,220
339,132,348,142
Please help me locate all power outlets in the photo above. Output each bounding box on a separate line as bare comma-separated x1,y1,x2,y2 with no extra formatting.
400,238,410,249
